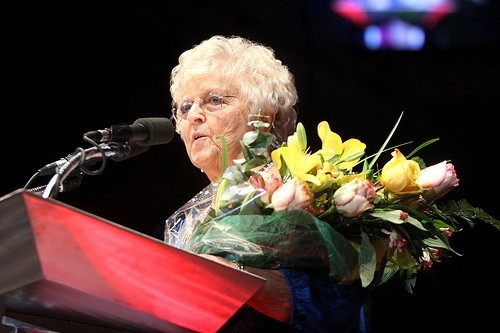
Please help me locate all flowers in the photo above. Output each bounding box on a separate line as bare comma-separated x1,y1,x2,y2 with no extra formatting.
190,110,500,296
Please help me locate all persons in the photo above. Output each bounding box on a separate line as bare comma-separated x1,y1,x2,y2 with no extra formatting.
169,36,366,333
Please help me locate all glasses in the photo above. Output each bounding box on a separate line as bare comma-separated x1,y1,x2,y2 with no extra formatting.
172,91,237,116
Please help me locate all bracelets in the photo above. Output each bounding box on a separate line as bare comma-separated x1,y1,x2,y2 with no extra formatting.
237,264,243,271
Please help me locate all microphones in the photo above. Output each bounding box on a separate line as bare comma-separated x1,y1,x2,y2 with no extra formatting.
28,177,81,195
86,118,174,146
38,143,149,177
